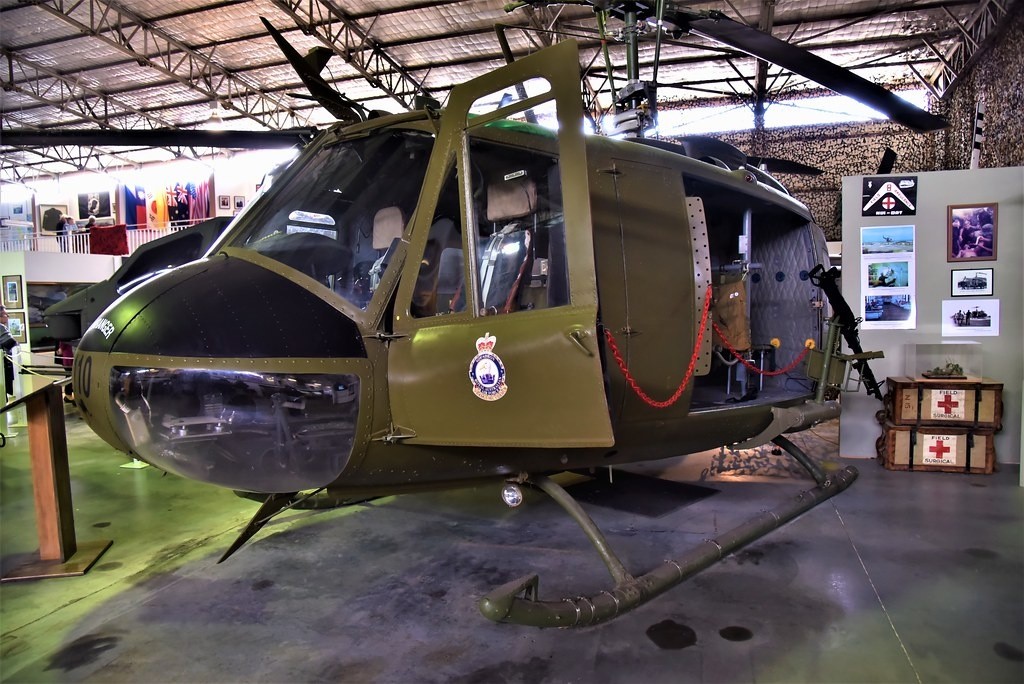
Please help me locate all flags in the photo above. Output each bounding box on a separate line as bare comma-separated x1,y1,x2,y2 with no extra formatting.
119,171,212,231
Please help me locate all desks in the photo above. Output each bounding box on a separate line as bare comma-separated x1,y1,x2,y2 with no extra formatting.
0,374,113,582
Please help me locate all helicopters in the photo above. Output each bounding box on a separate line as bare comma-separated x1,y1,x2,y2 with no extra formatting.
71,1,950,631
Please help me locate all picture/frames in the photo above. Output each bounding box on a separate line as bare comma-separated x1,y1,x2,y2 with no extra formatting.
4,312,27,343
39,204,68,237
2,275,23,309
947,203,998,262
951,268,994,297
219,196,230,209
234,196,245,209
233,211,240,216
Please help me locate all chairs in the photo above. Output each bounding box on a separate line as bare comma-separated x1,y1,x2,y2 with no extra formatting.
449,172,538,315
368,207,408,295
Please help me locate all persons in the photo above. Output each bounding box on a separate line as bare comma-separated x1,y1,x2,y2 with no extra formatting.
952,206,994,257
953,309,972,326
56,213,96,254
0,304,20,401
879,269,897,286
56,340,78,407
89,199,98,213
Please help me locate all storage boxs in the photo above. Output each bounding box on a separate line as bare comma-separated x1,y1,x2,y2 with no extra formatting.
875,377,1004,474
